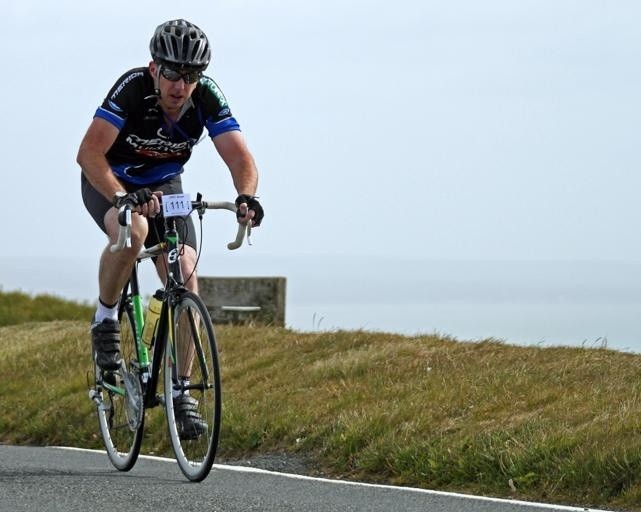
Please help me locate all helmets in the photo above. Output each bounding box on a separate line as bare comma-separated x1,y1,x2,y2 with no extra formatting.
149,19,212,71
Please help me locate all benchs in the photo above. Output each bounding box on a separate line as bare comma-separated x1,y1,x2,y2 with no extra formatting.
205,304,264,323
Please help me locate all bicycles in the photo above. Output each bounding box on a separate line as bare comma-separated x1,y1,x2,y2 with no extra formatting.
86,192,255,482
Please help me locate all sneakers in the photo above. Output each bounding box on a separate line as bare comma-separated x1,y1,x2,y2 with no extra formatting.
91,315,123,370
173,394,209,435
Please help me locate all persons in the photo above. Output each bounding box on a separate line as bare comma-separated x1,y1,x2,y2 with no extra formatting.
76,18,264,438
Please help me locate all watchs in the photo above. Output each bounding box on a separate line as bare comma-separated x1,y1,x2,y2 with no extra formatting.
112,191,128,208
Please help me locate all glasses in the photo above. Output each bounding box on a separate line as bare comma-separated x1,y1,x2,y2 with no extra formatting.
160,67,201,84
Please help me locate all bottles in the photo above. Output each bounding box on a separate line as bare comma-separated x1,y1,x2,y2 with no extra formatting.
140,288,165,345
